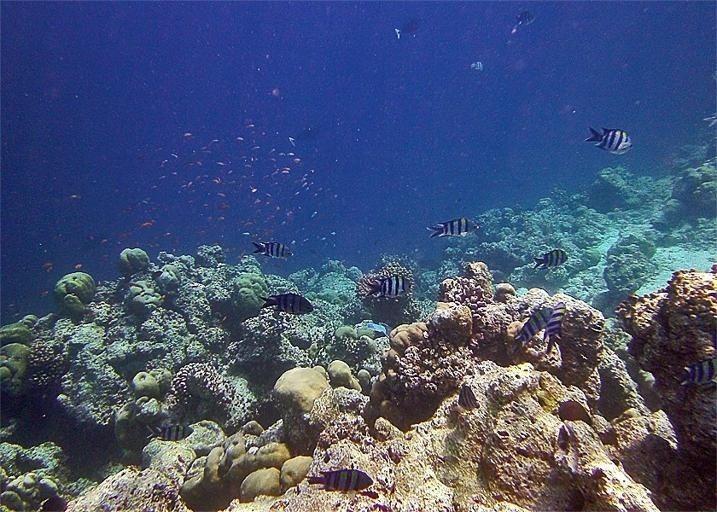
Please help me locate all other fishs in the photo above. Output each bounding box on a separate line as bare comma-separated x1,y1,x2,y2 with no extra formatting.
257,294,314,319
134,124,336,262
0,227,131,329
121,205,132,215
65,192,83,204
